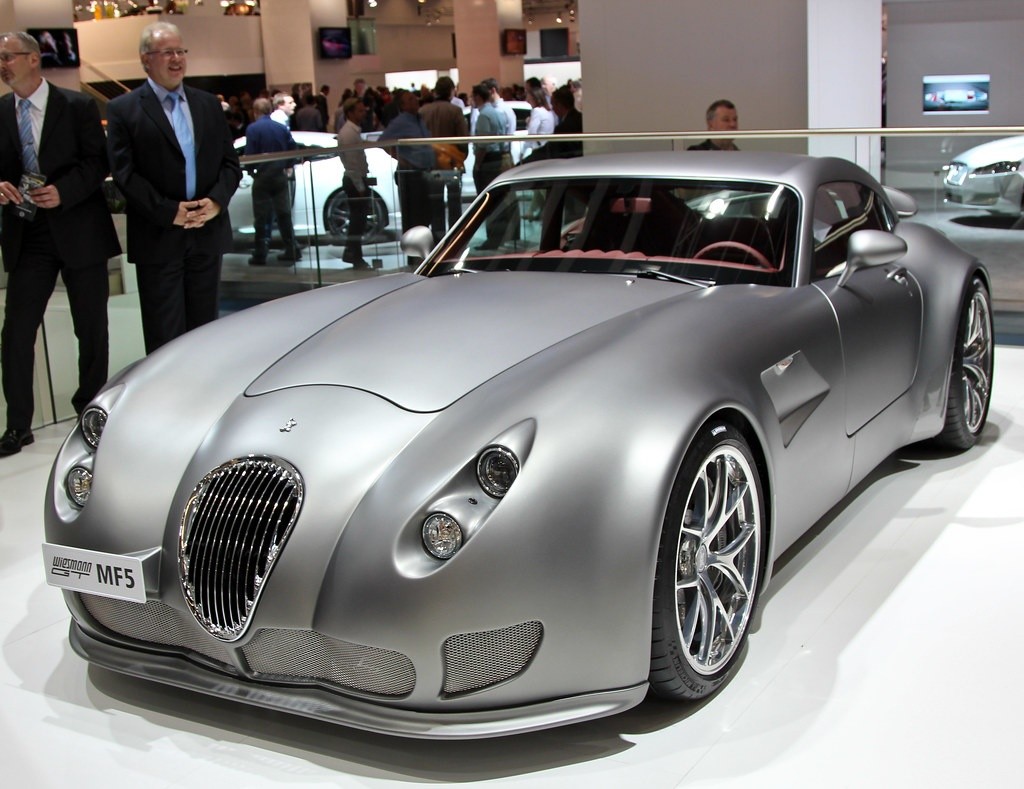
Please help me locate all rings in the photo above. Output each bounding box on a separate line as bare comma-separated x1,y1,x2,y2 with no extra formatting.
0,192,4,196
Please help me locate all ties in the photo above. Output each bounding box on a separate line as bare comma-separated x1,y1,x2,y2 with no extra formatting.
166,92,197,201
16,99,41,175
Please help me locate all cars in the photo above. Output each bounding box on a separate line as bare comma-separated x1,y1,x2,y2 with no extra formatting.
940,134,1024,218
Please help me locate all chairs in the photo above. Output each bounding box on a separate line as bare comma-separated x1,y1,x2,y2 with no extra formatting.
702,214,774,266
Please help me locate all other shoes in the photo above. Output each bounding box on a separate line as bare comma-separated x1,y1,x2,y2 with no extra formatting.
249,255,267,266
278,246,302,261
474,240,503,251
342,248,371,271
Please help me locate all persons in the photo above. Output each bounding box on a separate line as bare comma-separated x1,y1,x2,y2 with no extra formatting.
337,97,374,269
216,90,273,142
106,23,242,355
375,87,439,236
419,75,469,241
467,73,582,250
0,32,123,459
331,75,437,132
674,98,744,201
246,96,303,263
270,83,331,133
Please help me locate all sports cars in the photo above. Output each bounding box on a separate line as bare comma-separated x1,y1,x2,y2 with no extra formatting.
43,149,997,740
227,103,535,249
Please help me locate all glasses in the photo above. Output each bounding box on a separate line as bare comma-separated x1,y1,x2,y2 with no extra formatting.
144,46,189,61
0,52,32,62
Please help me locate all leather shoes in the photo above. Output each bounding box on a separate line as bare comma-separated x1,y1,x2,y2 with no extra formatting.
0,426,34,455
